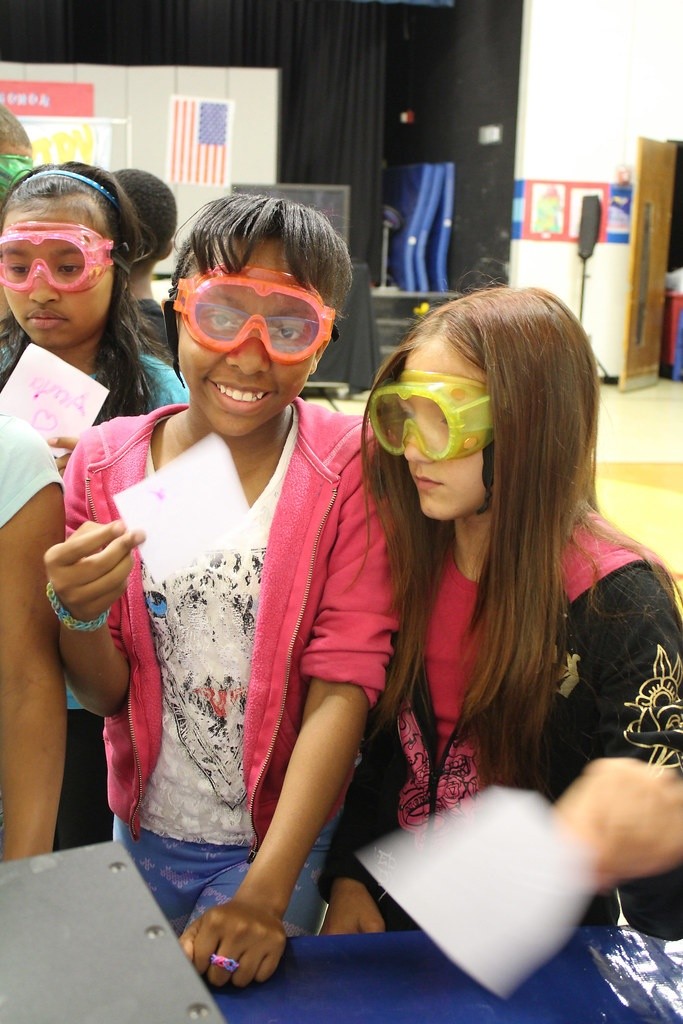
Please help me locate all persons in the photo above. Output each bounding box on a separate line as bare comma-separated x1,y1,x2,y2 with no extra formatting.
2,158,192,476
0,406,66,864
0,102,34,322
317,285,683,943
38,193,398,988
112,168,174,368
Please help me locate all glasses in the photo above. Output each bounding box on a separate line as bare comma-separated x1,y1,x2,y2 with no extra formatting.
368,370,497,456
0,221,115,292
172,263,336,366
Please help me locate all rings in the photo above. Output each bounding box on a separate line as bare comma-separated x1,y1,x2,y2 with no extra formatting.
210,954,240,972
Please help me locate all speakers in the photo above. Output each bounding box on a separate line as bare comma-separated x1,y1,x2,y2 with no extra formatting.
578,196,601,261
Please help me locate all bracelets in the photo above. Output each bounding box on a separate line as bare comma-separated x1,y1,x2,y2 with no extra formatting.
46,583,111,633
